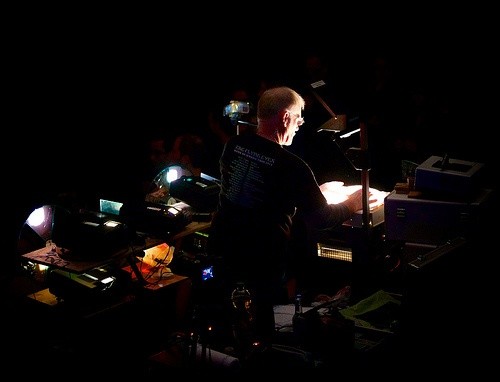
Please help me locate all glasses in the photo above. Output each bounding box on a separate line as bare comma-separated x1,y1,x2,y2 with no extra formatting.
288,111,304,126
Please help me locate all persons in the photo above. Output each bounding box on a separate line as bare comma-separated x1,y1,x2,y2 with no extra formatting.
143,51,339,188
206,87,365,304
